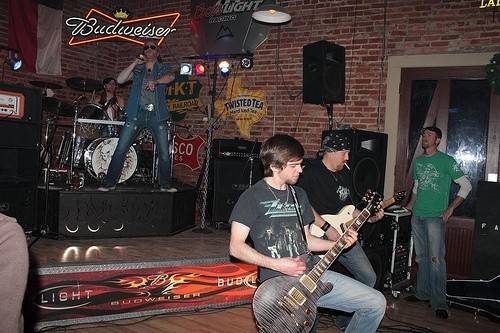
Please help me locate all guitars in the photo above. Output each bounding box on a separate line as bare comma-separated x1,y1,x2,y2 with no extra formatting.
252,188,382,333
308,189,408,260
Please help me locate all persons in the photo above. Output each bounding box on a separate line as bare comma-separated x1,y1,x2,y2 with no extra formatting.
298,134,384,333
0,212,29,333
89,77,125,118
406,126,472,320
97,42,177,193
228,134,387,333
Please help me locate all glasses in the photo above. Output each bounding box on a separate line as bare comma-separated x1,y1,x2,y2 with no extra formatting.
143,45,157,51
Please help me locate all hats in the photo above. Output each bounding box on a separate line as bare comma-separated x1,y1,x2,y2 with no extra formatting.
104,77,117,84
322,133,351,152
418,126,442,137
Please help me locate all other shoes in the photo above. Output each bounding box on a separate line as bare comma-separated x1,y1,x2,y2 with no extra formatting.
404,295,430,303
161,187,178,192
436,308,448,320
97,185,116,191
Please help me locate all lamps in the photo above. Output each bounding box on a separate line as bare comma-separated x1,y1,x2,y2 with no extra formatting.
180,63,193,76
194,62,208,74
240,55,253,69
252,0,292,23
219,57,233,73
4,55,23,70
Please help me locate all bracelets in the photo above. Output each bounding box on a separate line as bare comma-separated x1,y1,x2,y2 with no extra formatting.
320,222,331,232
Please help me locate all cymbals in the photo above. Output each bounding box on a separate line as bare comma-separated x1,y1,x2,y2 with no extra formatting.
42,97,75,117
66,77,103,91
29,81,63,90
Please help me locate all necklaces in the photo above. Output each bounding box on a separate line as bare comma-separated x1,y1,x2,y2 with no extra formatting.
263,177,290,212
331,173,338,183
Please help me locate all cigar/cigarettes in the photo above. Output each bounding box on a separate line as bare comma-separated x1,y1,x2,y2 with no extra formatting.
344,162,351,170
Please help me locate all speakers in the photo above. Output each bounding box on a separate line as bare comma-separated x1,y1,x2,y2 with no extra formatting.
214,138,267,222
321,129,388,206
470,180,500,297
0,84,43,230
302,40,345,104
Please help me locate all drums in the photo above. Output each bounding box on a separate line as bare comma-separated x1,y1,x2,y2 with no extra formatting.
56,132,96,170
99,123,122,137
74,103,109,139
82,137,139,185
